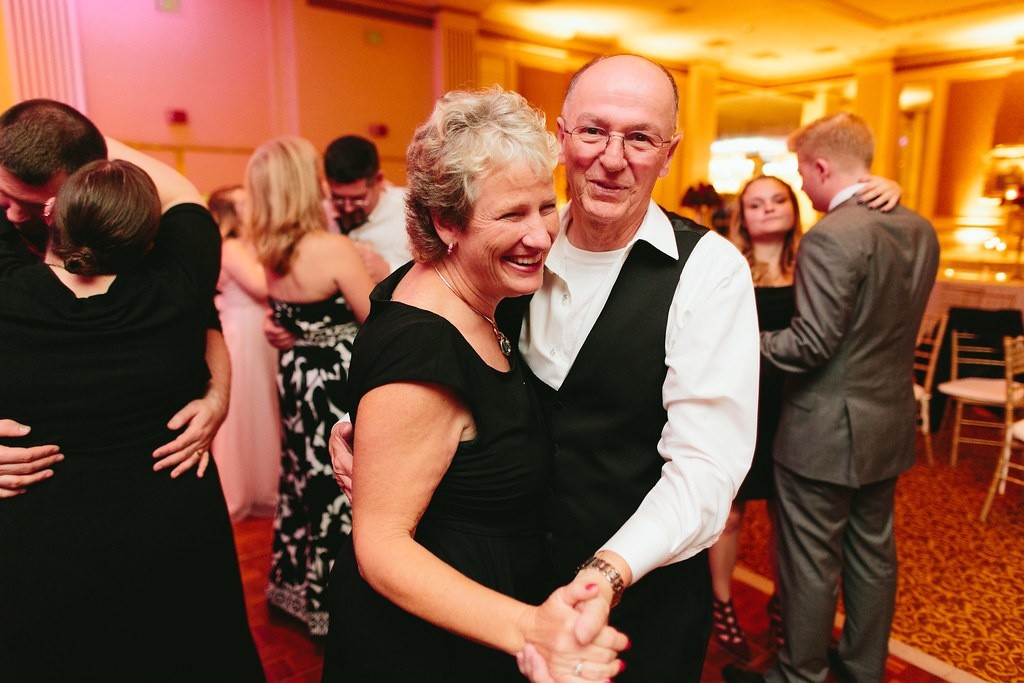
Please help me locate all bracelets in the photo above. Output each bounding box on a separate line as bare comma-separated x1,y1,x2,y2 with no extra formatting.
575,557,623,607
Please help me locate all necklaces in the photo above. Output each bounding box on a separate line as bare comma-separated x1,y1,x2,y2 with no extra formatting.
433,261,512,356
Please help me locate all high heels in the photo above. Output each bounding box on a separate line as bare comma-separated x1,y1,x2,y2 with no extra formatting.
766,594,786,645
712,594,752,663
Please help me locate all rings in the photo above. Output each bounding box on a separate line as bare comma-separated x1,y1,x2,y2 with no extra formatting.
195,451,201,456
574,660,585,674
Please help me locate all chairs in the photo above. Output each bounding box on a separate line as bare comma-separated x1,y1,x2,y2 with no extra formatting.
914,312,1024,524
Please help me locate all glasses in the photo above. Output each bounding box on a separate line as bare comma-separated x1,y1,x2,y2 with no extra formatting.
331,186,373,207
563,126,673,155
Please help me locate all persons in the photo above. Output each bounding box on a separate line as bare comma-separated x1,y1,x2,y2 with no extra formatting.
0,159,266,683
217,137,375,646
0,97,232,498
207,182,280,522
329,52,762,683
263,136,420,351
709,175,905,661
322,82,632,683
721,112,940,683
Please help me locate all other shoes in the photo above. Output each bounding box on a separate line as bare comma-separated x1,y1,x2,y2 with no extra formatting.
722,665,765,683
